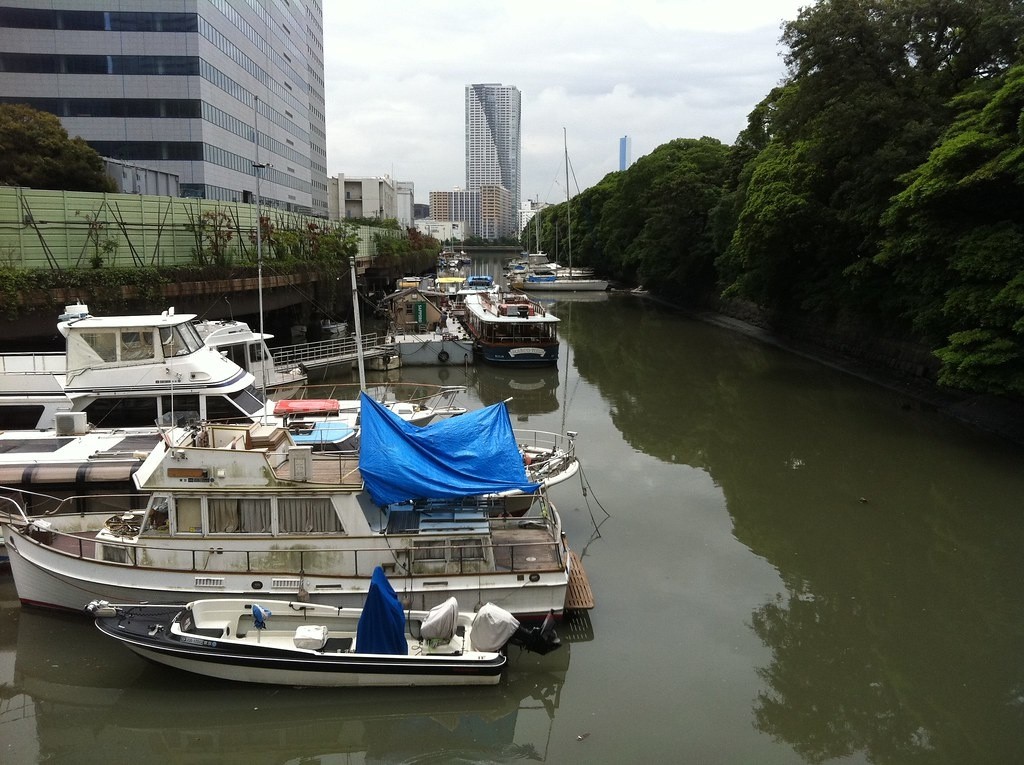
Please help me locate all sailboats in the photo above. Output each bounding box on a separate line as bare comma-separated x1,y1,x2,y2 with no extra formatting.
436,223,473,270
503,122,608,292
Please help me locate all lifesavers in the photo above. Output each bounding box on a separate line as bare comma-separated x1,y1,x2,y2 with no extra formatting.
438,351,449,362
383,355,390,364
438,368,449,380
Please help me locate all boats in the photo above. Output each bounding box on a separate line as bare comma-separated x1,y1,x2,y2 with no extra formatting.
1,418,595,625
630,287,648,295
321,318,348,334
383,276,563,369
85,599,564,689
1,301,580,515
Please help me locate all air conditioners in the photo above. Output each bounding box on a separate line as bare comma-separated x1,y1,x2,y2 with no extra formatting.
56,412,87,434
